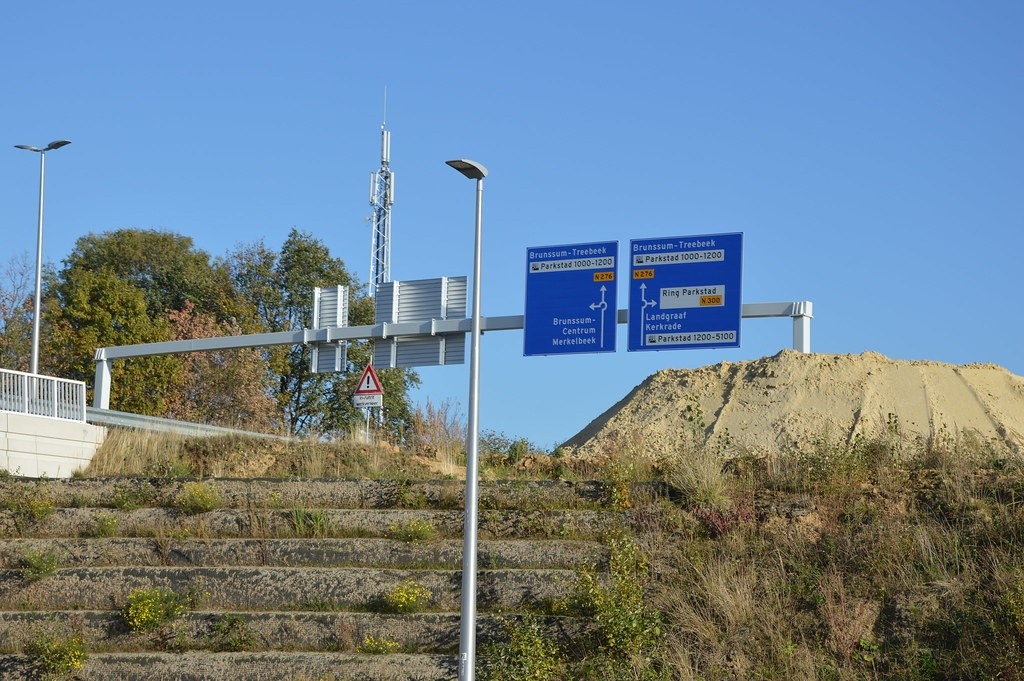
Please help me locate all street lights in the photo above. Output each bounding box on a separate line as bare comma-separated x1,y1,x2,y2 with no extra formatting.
14,138,72,403
444,158,489,681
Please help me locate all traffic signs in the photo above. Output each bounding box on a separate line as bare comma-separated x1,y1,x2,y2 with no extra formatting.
522,239,621,358
626,231,744,352
